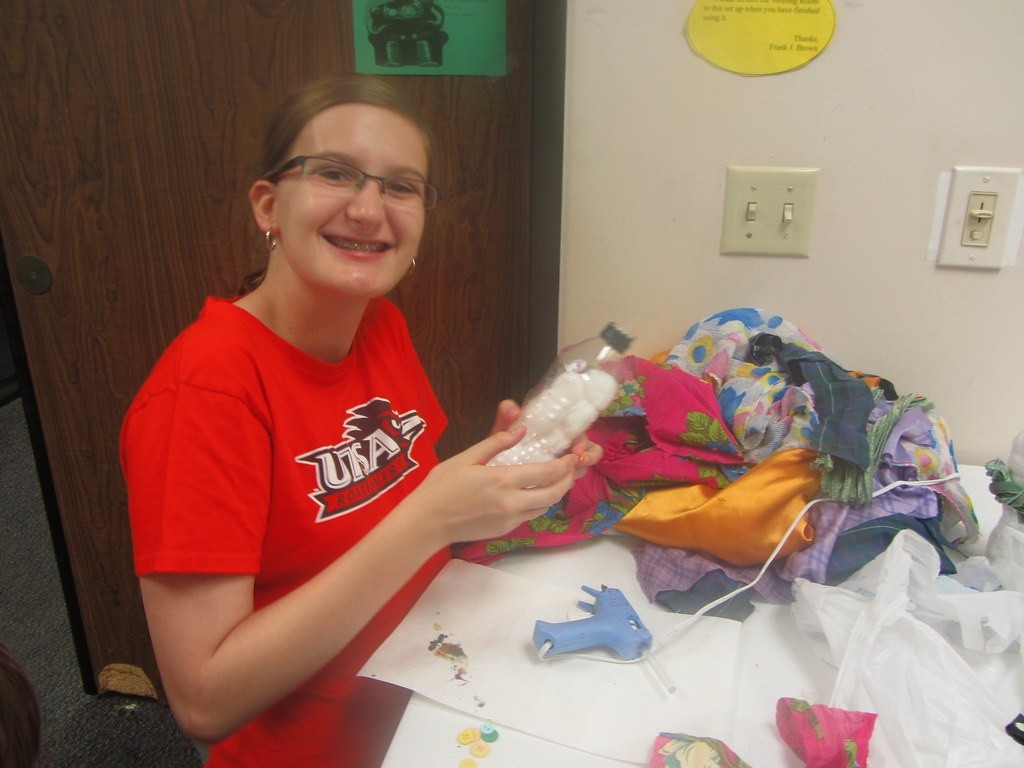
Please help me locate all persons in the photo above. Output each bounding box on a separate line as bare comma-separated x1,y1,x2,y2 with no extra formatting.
116,74,607,768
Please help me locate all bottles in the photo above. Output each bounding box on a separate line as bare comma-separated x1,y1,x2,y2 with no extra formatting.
484,321,643,467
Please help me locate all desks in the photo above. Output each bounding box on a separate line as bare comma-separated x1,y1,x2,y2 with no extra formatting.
335,378,1024,768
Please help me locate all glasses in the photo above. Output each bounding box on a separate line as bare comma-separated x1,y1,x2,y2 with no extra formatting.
264,156,440,212
